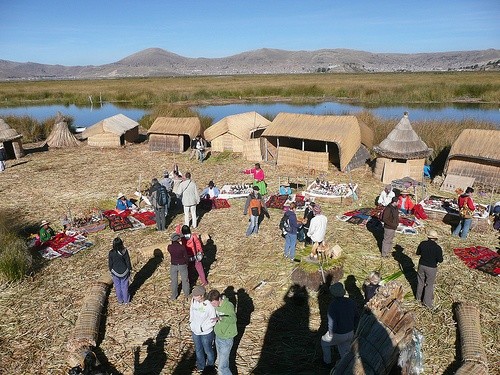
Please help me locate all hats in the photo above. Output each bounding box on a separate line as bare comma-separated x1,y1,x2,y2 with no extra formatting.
207,181,215,186
314,205,322,212
117,193,124,199
253,186,260,190
171,234,179,241
192,286,206,297
427,230,438,238
40,220,49,227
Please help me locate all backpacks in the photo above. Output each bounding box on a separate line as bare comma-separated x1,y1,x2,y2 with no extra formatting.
280,213,290,237
157,186,168,206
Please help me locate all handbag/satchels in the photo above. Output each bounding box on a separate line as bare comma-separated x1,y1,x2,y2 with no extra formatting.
252,207,258,216
459,197,475,219
297,229,306,242
173,199,182,208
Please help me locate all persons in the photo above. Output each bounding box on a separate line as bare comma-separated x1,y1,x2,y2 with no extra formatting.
108,235,132,304
201,181,220,199
362,272,381,301
452,187,475,239
137,163,200,232
190,135,207,162
397,189,415,214
79,353,100,375
180,225,208,285
382,197,400,258
190,287,238,375
320,282,356,365
167,233,189,299
40,219,55,242
487,205,500,232
116,193,146,229
280,202,328,263
378,185,396,209
239,163,270,236
416,228,443,305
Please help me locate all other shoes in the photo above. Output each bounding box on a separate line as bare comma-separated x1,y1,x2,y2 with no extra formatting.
290,258,294,262
452,234,458,238
